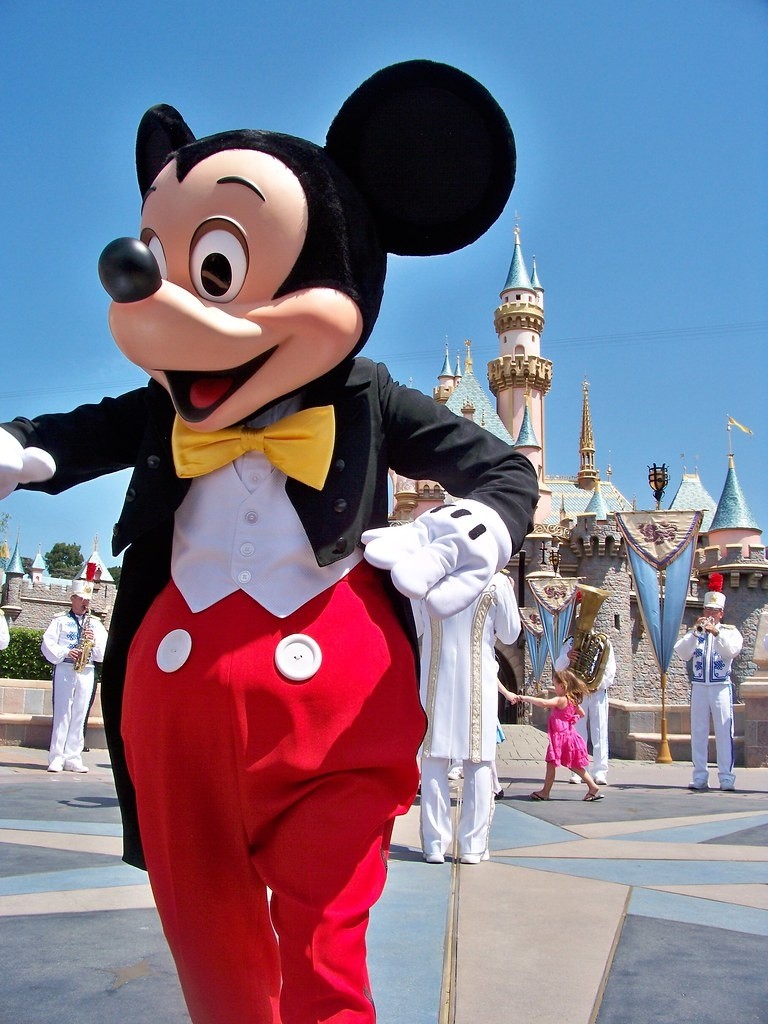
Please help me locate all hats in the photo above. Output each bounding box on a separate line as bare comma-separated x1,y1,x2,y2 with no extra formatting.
70,562,97,600
702,572,726,609
575,591,582,619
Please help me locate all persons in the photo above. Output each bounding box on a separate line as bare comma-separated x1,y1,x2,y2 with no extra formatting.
41,579,108,773
517,669,605,801
555,591,616,783
674,591,744,791
48,662,97,752
0,607,10,651
492,629,517,801
410,572,521,863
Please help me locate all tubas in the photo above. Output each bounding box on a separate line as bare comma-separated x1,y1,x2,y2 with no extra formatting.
567,582,612,691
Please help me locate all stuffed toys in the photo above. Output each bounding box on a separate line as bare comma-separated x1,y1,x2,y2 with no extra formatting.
0,59,539,1024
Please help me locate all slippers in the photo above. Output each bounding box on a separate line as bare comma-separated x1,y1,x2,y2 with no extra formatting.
529,792,549,801
583,793,604,801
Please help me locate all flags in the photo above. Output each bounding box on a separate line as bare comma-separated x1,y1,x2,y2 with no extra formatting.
728,416,752,435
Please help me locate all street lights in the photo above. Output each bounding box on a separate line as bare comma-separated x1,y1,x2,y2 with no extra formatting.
646,461,673,764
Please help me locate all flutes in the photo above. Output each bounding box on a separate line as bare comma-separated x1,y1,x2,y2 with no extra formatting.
696,616,715,634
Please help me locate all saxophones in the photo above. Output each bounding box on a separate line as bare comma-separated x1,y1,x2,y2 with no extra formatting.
73,606,94,673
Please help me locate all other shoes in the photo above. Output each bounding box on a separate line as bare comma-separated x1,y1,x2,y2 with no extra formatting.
48,762,63,771
569,774,583,784
422,852,445,863
720,779,735,790
461,849,489,863
688,778,708,788
64,764,89,772
448,768,465,780
82,747,89,752
594,776,607,785
